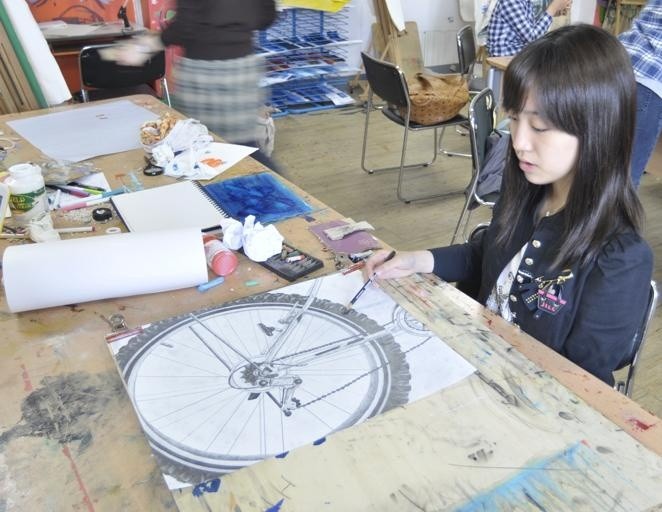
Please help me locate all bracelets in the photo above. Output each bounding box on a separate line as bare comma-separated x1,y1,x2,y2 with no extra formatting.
136,40,156,56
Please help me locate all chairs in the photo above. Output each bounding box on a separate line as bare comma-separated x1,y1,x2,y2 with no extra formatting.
358,23,500,247
75,42,172,111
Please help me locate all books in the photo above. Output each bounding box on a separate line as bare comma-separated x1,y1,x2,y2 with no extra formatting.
111,180,230,237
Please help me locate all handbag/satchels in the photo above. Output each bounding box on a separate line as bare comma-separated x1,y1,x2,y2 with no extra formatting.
397,67,471,128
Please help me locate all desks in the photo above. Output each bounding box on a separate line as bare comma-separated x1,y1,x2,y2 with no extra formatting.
1,91,659,512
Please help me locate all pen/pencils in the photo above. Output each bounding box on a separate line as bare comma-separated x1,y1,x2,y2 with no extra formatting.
55,226,96,233
344,250,396,316
46,181,125,213
279,244,306,263
198,277,225,294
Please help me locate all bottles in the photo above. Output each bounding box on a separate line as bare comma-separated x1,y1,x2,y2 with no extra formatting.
6,164,47,224
200,231,236,278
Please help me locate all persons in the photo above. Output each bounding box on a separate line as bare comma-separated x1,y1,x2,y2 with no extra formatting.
486,0,573,110
363,24,655,388
612,0,662,193
112,1,285,178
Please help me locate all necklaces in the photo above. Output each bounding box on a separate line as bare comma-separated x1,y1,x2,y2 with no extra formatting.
544,204,564,216
